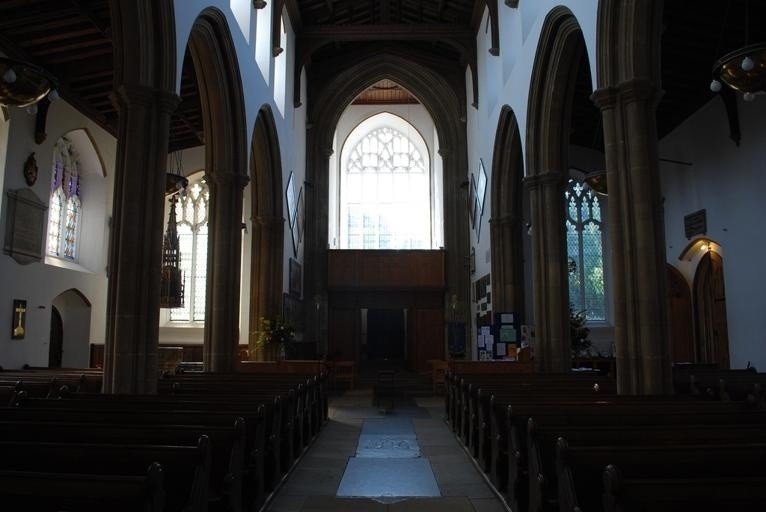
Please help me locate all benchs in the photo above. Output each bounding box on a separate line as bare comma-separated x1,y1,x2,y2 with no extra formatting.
441,355,766,512
0,364,330,512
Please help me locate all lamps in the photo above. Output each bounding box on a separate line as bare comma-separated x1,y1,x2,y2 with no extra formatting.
709,0,766,103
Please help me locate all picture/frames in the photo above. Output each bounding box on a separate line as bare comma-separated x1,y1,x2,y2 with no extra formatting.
289,258,301,297
11,299,27,340
